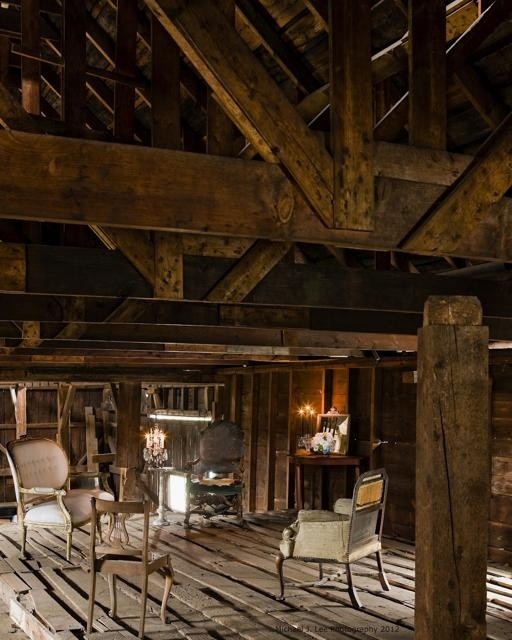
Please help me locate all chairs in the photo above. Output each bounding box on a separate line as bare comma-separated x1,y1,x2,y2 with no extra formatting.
0,419,390,640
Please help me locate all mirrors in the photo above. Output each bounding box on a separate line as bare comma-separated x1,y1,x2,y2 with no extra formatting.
316,414,350,458
